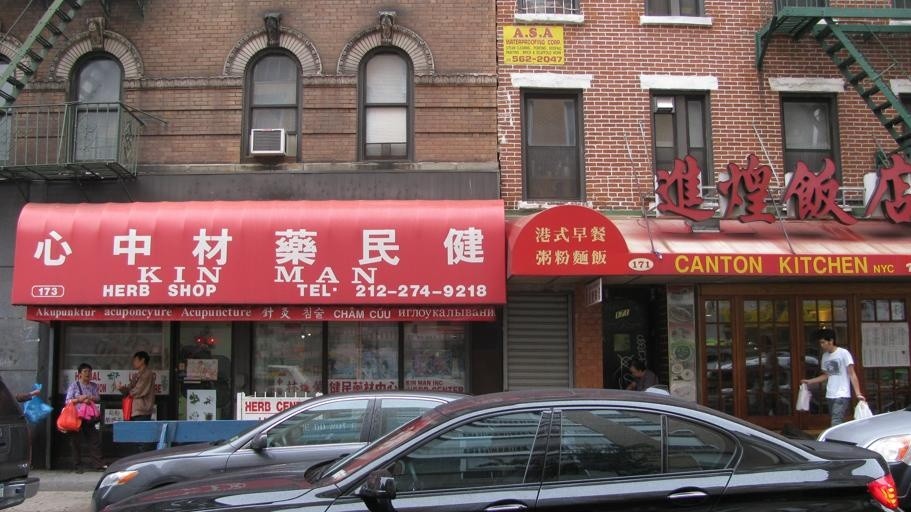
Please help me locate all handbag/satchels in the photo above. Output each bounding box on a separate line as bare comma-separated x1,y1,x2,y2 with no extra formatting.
76,399,101,425
795,382,811,410
120,387,133,421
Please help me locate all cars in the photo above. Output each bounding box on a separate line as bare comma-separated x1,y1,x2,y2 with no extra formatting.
704,348,818,385
815,404,910,512
0,379,40,510
91,390,470,509
99,387,899,512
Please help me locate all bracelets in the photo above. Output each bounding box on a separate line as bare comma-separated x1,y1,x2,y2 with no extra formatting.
856,392,863,396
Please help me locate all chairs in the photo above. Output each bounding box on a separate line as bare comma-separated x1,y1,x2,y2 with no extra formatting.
668,429,703,473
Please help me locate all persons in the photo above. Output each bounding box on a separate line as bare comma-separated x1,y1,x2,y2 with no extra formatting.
17,389,40,402
629,359,660,391
116,350,156,452
800,327,866,427
65,363,108,474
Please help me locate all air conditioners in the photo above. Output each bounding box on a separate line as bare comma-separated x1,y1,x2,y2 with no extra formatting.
249,128,288,157
